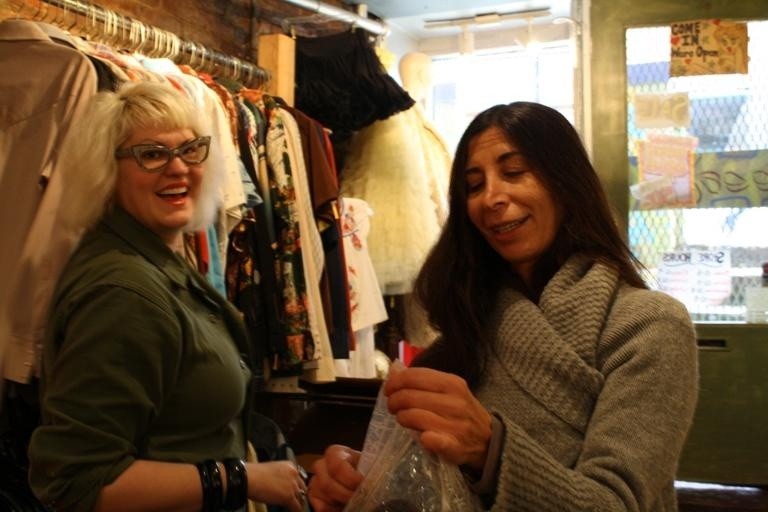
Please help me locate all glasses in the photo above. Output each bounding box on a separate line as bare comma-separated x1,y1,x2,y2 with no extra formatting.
115,136,211,174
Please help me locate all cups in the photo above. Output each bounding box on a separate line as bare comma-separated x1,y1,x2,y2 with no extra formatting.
742,285,768,324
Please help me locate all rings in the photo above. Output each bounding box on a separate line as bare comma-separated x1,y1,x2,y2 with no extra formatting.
298,489,306,506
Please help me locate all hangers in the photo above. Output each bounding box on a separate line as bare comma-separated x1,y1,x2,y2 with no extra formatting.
280,0,358,38
14,0,272,94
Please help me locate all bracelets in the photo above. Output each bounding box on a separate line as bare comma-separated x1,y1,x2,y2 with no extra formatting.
193,456,223,511
224,457,248,511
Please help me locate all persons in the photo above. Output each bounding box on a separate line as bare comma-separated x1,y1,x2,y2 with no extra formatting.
307,101,701,510
24,75,307,511
399,53,456,233
